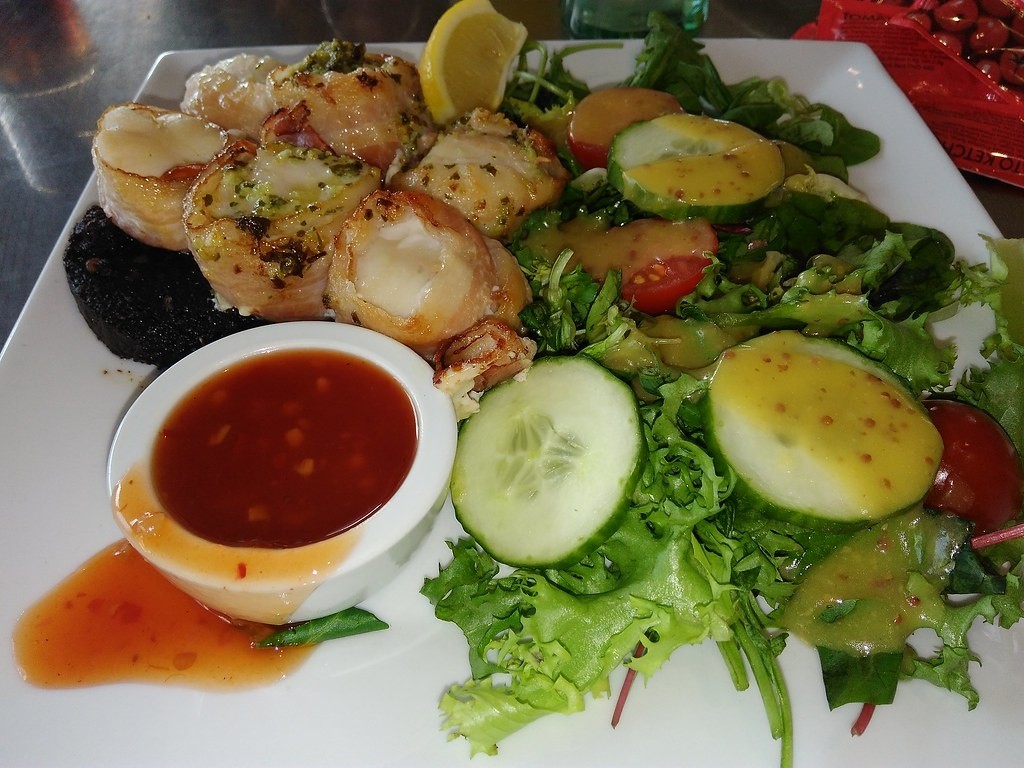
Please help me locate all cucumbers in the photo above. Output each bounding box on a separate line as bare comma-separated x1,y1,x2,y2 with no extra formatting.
606,114,787,219
448,355,649,570
703,330,947,529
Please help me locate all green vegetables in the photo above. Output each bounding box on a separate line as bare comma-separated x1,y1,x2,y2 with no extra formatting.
256,20,1024,768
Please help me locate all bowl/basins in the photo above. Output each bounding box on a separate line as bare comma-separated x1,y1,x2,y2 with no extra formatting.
106,320,457,623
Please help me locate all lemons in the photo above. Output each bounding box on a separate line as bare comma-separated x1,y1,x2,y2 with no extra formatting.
420,0,528,124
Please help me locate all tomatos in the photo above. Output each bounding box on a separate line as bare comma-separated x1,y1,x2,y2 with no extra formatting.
589,219,719,313
569,87,687,169
915,400,1023,535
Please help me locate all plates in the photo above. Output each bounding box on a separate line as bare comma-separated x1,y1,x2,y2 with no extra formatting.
0,36,1024,768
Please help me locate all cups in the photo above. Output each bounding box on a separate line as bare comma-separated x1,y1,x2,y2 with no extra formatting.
560,0,710,44
0,0,98,98
